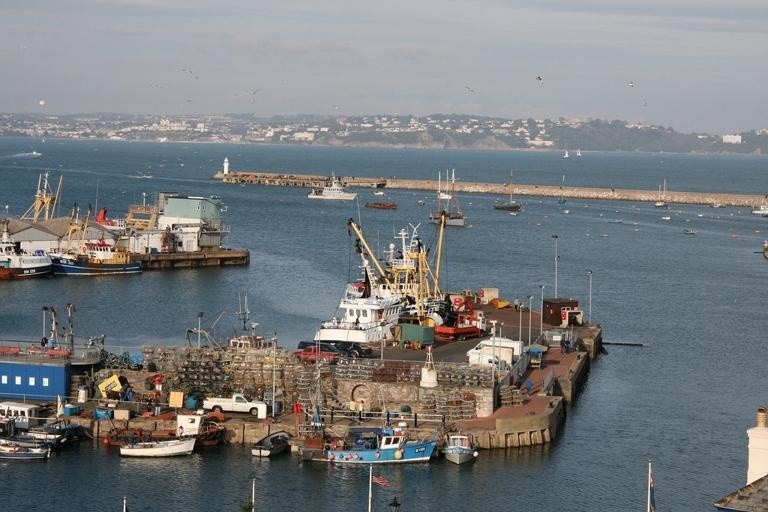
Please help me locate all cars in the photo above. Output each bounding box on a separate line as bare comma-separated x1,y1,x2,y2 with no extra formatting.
293,342,372,364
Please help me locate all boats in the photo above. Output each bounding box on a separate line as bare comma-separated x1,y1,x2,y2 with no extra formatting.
313,222,424,342
102,414,226,458
494,200,524,211
227,336,277,351
0,233,143,278
0,401,83,460
751,205,768,217
655,202,669,207
308,183,358,201
251,424,478,464
364,201,398,210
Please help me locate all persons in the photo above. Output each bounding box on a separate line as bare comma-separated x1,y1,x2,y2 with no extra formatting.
513,297,520,312
358,400,364,421
349,398,357,419
145,393,160,416
176,426,184,441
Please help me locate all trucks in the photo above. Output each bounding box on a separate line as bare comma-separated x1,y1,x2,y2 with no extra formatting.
435,316,487,341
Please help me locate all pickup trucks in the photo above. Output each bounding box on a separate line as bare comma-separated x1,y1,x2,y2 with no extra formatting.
202,392,267,416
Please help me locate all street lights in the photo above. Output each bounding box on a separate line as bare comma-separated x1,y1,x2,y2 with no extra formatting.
518,232,593,356
490,319,505,387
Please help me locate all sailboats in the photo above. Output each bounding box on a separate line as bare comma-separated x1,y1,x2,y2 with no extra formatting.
563,149,569,159
429,169,465,226
577,148,582,158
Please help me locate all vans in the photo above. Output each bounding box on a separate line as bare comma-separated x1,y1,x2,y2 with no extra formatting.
466,340,523,362
